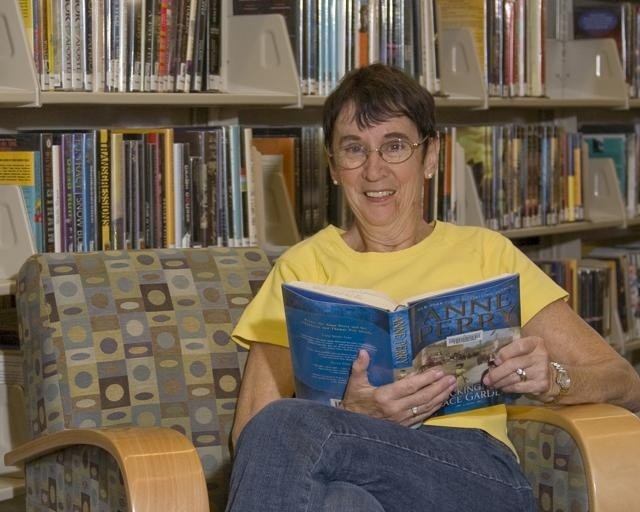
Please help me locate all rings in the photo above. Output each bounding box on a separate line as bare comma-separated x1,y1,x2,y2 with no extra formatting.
513,367,526,381
410,406,418,416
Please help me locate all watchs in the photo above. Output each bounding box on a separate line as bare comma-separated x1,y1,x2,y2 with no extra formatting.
545,361,572,405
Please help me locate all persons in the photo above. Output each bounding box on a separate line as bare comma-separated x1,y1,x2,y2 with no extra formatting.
229,62,639,512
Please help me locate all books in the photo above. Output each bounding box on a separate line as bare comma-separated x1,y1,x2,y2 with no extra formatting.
280,269,523,418
0,1,260,248
231,0,640,347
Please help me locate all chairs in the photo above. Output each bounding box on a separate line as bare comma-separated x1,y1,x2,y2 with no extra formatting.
4,245,639,512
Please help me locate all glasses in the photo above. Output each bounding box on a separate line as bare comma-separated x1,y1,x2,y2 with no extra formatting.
322,132,433,171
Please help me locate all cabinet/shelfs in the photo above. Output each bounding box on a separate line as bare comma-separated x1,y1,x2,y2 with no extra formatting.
0,1,640,512
